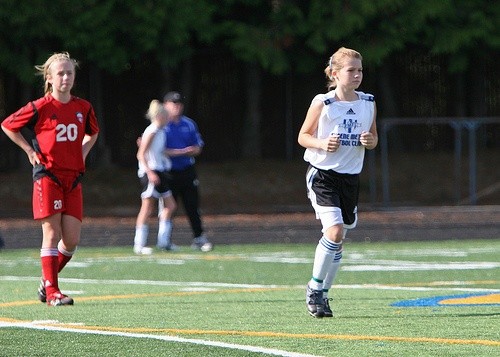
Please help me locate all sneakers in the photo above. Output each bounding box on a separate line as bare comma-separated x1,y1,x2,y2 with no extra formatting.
46,291,74,307
39,278,46,303
323,297,333,317
306,281,326,318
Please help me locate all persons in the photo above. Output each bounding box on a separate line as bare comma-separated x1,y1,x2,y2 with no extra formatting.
137,92,213,252
133,99,176,255
1,52,99,306
298,47,378,318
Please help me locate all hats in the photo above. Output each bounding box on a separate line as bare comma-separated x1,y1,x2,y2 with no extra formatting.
164,90,188,102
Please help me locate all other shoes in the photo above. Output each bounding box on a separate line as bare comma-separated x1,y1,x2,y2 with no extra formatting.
157,242,178,250
192,236,212,251
133,246,154,255
